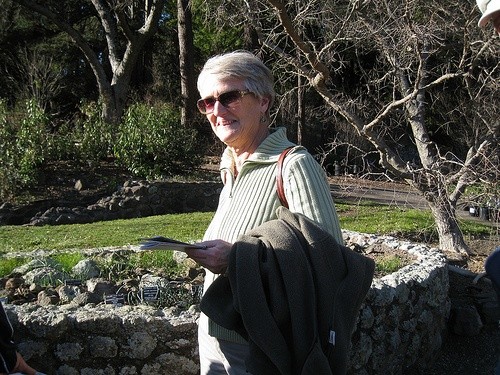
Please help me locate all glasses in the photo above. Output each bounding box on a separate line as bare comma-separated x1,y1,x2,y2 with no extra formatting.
197,89,254,115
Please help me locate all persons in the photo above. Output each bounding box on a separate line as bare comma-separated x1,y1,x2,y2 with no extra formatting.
184,50,343,375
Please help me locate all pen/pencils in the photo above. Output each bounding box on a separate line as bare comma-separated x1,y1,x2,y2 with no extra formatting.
186,245,208,258
